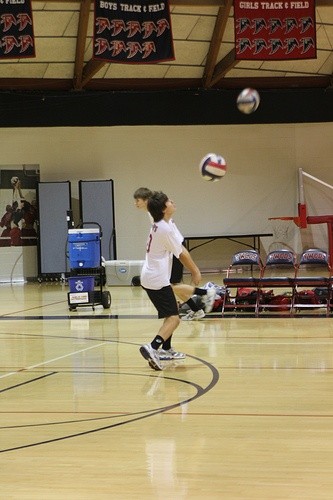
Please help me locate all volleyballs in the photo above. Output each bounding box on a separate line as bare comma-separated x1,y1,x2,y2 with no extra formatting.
199,153,227,181
10,177,19,184
236,88,261,115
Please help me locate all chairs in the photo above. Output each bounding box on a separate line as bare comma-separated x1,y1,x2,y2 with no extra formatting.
222,250,264,318
291,248,332,318
256,250,297,317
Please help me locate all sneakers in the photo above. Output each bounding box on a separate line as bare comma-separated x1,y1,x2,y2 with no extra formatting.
139,343,163,370
158,346,187,360
181,308,205,321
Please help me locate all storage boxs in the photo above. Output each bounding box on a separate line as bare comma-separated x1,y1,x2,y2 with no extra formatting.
68,228,100,270
68,276,94,293
103,259,146,286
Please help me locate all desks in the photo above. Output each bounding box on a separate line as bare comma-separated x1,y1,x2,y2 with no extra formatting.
183,233,273,271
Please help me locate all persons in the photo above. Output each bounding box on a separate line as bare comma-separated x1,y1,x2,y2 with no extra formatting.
0,182,38,237
132,185,216,372
